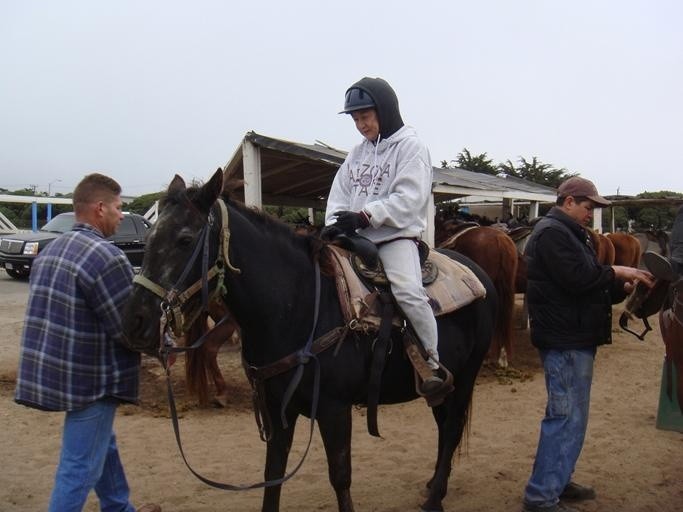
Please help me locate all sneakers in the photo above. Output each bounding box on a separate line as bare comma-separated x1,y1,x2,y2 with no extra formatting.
523,500,580,512
136,503,161,511
642,250,683,291
559,481,596,499
420,369,447,406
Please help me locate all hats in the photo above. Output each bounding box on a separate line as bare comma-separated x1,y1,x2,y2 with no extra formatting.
557,177,612,208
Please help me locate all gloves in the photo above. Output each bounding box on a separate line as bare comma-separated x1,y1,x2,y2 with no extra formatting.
319,210,365,241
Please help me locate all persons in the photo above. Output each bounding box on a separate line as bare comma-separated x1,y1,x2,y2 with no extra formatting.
13,172,179,512
322,75,444,396
522,177,653,511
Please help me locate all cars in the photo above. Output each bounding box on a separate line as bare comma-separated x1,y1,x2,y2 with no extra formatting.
0,210,154,280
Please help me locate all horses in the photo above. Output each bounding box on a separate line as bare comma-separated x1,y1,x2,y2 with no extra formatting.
601,232,642,269
597,234,616,265
120,166,501,512
631,229,669,257
619,272,683,415
183,298,239,408
435,220,519,370
481,223,600,329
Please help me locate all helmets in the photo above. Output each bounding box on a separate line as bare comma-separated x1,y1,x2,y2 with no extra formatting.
339,88,376,115
